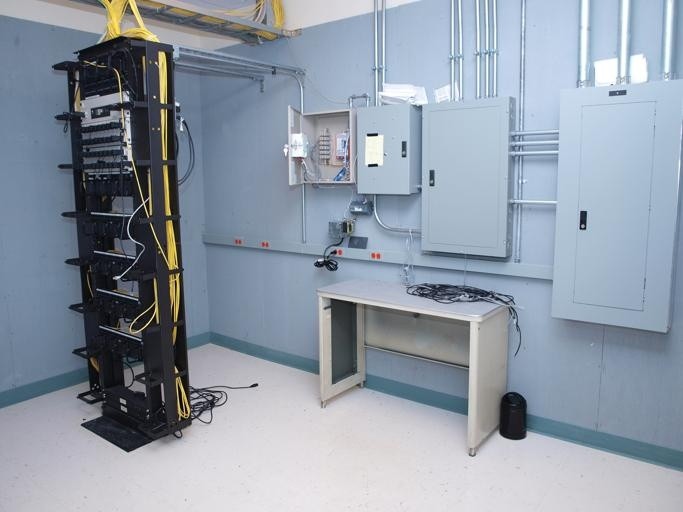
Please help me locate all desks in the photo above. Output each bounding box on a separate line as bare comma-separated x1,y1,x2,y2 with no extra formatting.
315,278,513,457
